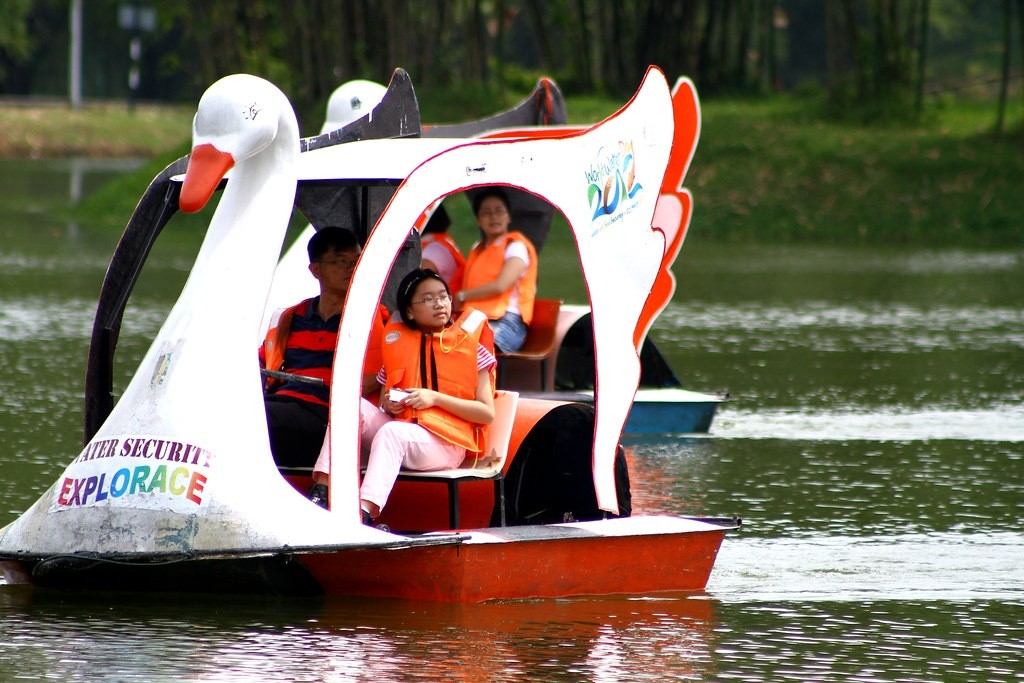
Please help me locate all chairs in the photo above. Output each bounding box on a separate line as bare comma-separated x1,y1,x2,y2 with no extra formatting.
492,299,562,392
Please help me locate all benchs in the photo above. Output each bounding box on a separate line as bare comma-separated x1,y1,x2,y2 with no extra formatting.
275,389,518,529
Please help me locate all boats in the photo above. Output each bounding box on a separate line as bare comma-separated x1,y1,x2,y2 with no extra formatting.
257,75,730,441
0,66,741,613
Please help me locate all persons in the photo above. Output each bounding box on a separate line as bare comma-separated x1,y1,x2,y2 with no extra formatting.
454,187,538,353
421,205,465,298
306,267,498,527
259,225,390,467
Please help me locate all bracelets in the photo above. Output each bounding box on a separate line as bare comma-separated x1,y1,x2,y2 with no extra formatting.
459,292,464,301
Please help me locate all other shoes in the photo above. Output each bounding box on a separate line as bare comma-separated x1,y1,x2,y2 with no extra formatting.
362,510,374,525
306,484,328,510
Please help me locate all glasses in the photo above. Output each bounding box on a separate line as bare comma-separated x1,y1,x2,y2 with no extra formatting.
313,257,357,268
480,206,508,218
411,294,454,308
